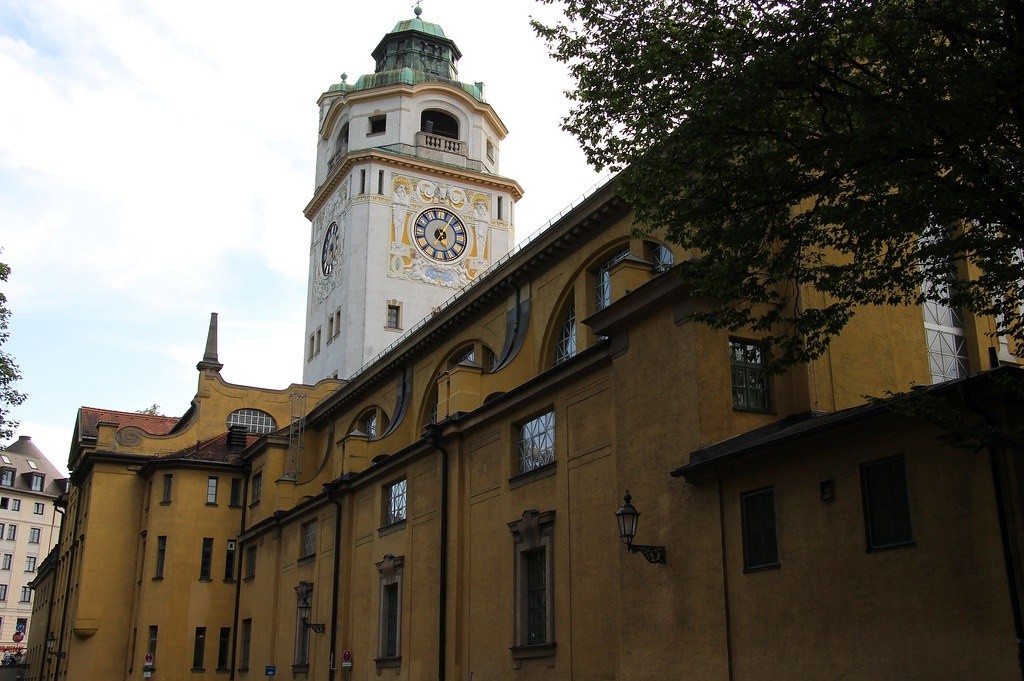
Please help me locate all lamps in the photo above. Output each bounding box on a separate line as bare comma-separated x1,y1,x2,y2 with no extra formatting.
613,490,666,565
297,595,326,633
45,630,66,659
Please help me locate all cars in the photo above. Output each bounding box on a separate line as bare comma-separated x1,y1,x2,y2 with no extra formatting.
2,654,17,665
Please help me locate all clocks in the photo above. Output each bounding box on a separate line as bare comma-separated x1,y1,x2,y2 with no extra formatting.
411,204,472,266
319,219,341,277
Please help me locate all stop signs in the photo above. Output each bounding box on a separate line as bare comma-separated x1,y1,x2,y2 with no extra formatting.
13,632,23,643
